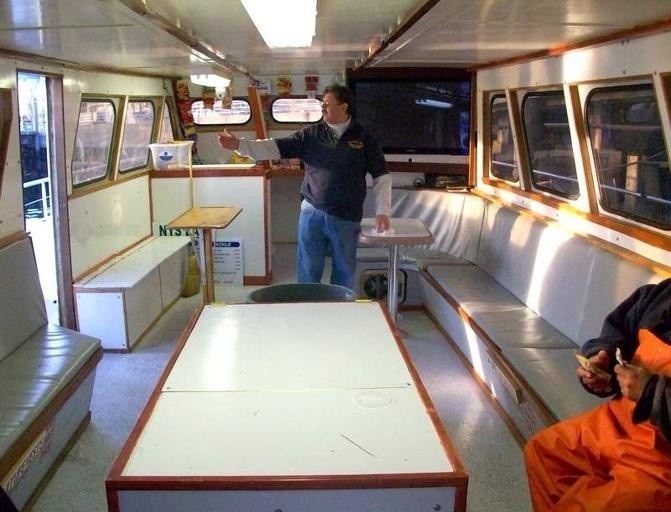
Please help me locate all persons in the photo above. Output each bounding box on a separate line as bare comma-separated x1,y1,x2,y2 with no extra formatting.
216,84,394,292
523,275,671,511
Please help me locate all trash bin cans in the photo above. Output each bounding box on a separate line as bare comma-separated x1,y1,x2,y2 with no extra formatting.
245,282,356,304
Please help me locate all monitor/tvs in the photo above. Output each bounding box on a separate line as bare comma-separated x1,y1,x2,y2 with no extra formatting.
345,67,473,166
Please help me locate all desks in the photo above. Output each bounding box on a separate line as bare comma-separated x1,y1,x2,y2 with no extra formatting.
164,206,242,302
356,217,434,340
106,299,469,510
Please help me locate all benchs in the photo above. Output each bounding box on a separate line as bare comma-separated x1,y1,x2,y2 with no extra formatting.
2,231,104,509
357,188,671,451
72,235,198,353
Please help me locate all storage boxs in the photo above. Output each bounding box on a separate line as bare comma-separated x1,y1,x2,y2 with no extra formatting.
148,139,194,171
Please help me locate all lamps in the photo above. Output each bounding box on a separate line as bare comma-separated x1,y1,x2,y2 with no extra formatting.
188,65,233,88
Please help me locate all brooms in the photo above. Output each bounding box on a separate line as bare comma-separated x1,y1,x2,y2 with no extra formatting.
180,145,201,297
